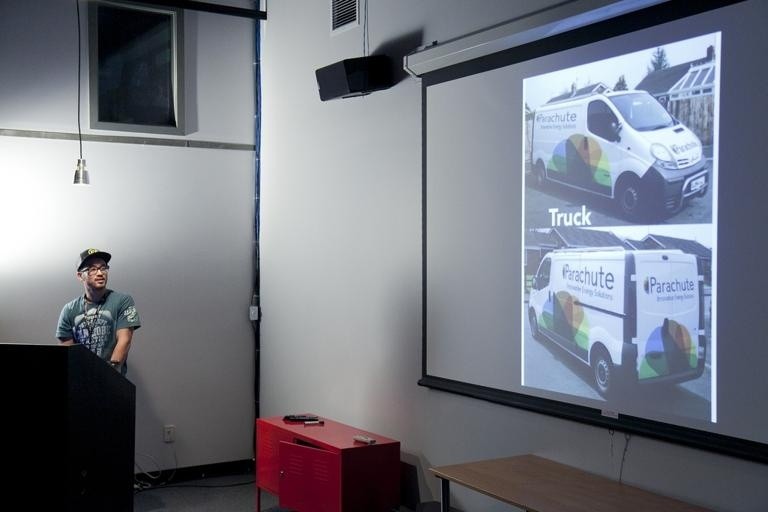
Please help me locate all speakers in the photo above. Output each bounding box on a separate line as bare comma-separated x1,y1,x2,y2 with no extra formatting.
316,57,385,101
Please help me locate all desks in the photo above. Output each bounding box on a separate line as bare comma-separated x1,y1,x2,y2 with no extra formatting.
428,453,713,512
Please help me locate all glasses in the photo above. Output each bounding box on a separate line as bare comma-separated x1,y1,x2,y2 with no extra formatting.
79,265,110,275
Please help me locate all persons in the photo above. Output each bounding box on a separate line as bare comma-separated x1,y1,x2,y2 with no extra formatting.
55,248,140,380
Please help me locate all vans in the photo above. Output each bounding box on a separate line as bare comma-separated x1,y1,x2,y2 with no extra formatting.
531,90,710,220
528,248,706,395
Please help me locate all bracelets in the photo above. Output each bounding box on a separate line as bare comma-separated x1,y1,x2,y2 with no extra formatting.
107,360,122,370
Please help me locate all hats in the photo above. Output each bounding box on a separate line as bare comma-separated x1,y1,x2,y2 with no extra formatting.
76,247,112,272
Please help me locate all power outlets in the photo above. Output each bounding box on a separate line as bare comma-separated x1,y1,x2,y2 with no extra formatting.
163,425,175,442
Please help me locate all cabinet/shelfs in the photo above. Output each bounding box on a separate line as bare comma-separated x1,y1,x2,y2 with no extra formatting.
253,413,400,512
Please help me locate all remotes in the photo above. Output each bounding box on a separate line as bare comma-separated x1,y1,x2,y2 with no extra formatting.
352,435,376,443
288,416,318,420
